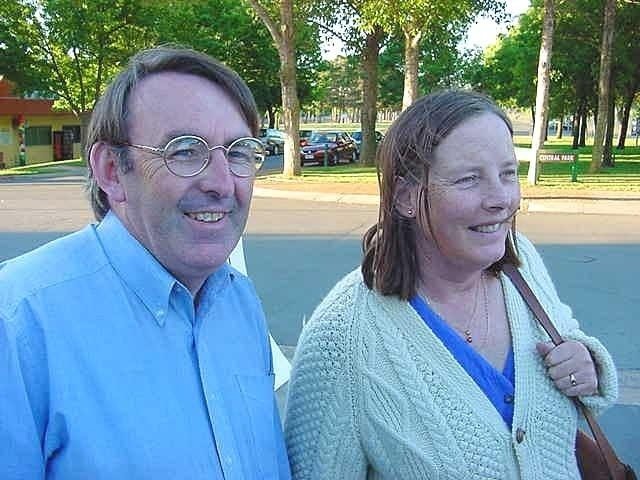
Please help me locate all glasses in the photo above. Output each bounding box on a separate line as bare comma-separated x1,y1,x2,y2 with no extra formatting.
106,135,267,178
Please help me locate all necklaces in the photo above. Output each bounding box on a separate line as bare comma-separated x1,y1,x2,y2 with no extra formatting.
421,273,490,352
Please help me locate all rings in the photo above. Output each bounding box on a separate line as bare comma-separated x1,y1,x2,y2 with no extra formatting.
570,374,577,387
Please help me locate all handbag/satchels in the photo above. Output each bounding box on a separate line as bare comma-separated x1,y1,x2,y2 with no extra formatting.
577,427,637,480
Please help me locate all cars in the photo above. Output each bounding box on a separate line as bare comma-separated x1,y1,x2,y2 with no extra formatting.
298,130,382,166
259,128,285,156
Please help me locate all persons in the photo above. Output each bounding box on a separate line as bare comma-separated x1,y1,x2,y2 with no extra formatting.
279,89,618,480
0,43,291,480
